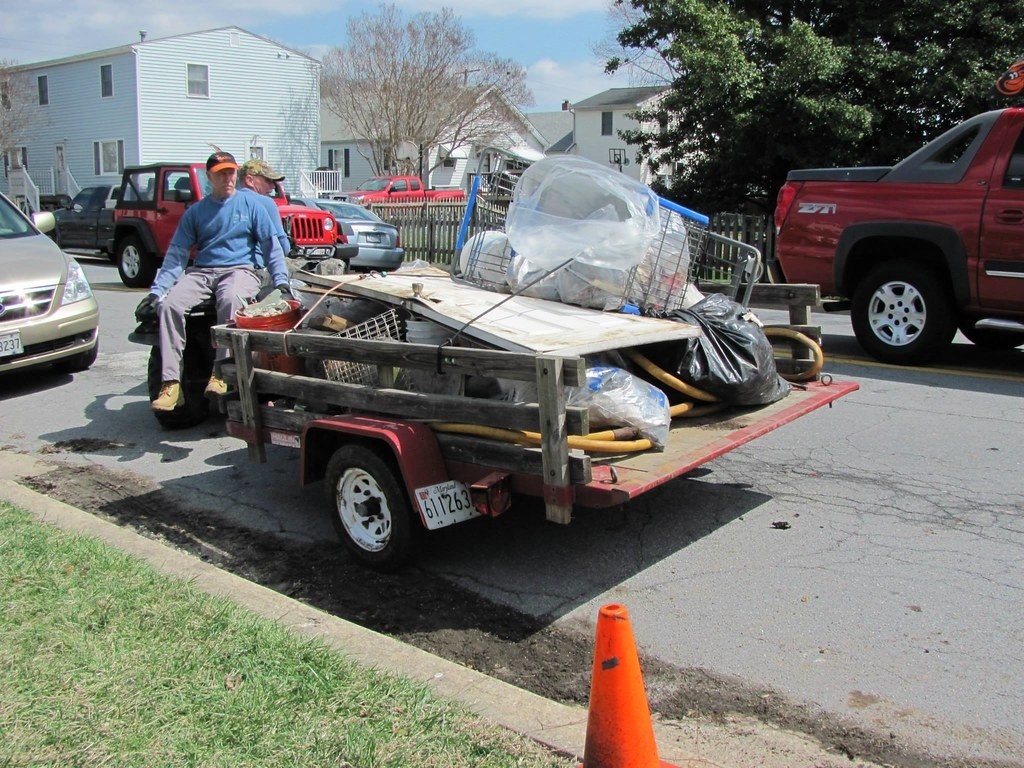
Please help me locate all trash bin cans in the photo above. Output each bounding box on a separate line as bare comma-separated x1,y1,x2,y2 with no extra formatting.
234,299,305,377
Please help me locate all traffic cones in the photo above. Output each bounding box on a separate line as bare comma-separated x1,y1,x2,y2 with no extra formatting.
576,606,681,768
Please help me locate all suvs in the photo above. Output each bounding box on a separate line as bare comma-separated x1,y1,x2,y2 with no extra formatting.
114,162,359,295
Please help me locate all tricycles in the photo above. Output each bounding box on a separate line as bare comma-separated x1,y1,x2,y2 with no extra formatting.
146,215,867,575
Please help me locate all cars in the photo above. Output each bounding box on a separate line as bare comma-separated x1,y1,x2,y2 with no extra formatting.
0,193,100,372
287,197,405,272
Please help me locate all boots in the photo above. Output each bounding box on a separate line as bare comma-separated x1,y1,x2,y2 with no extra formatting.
205,365,227,396
152,382,184,410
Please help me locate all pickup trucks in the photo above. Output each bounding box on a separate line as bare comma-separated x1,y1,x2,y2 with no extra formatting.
44,184,149,262
773,108,1023,366
331,176,465,201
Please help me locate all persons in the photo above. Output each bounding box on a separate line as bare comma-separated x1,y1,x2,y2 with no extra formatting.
134,152,292,413
238,159,291,257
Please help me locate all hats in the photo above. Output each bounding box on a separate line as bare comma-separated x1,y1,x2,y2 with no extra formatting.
206,152,240,173
240,160,285,182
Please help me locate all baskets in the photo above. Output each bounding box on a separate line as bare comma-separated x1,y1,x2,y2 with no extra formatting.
322,309,416,392
